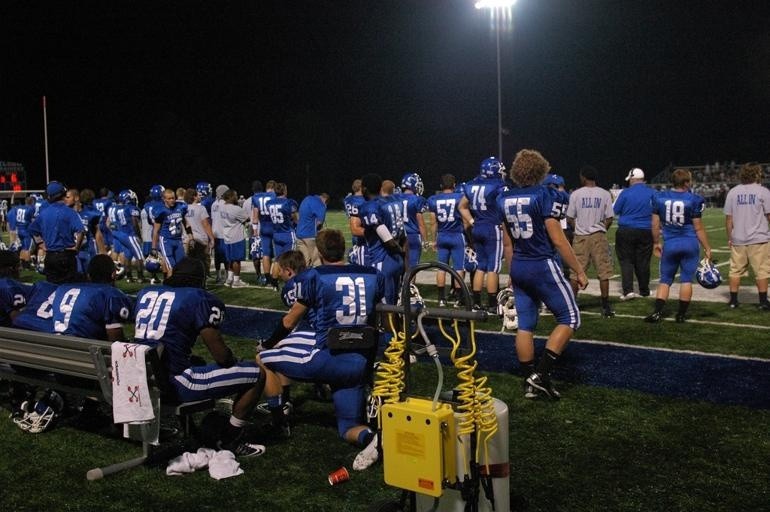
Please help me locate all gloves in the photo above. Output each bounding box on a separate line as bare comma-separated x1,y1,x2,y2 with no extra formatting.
150,248,159,259
256,338,272,352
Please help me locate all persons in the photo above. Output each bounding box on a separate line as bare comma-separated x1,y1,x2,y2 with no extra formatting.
258,228,381,472
498,150,588,400
1,249,265,456
0,147,770,322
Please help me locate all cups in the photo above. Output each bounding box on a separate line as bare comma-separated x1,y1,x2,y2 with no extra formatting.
328,466,350,486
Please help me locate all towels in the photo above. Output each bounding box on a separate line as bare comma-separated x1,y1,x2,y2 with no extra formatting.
108,341,162,447
165,447,245,481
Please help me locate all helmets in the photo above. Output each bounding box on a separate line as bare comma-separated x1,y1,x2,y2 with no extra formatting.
146,257,161,273
197,183,212,196
118,191,130,202
15,388,63,433
481,157,507,180
696,257,723,290
401,172,425,197
497,288,518,330
454,182,467,193
153,185,165,197
465,247,478,272
543,173,564,186
250,236,264,258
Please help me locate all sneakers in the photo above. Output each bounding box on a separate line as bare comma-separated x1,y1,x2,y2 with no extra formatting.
438,288,499,316
727,299,769,310
352,431,379,470
644,310,686,325
523,367,561,403
217,274,278,290
600,302,614,319
619,292,637,303
256,400,294,442
216,441,266,459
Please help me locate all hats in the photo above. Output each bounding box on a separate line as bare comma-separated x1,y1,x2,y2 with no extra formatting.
216,185,229,196
47,182,63,196
625,168,644,182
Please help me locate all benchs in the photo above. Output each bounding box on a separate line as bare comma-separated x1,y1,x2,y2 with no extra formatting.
0,326,218,482
